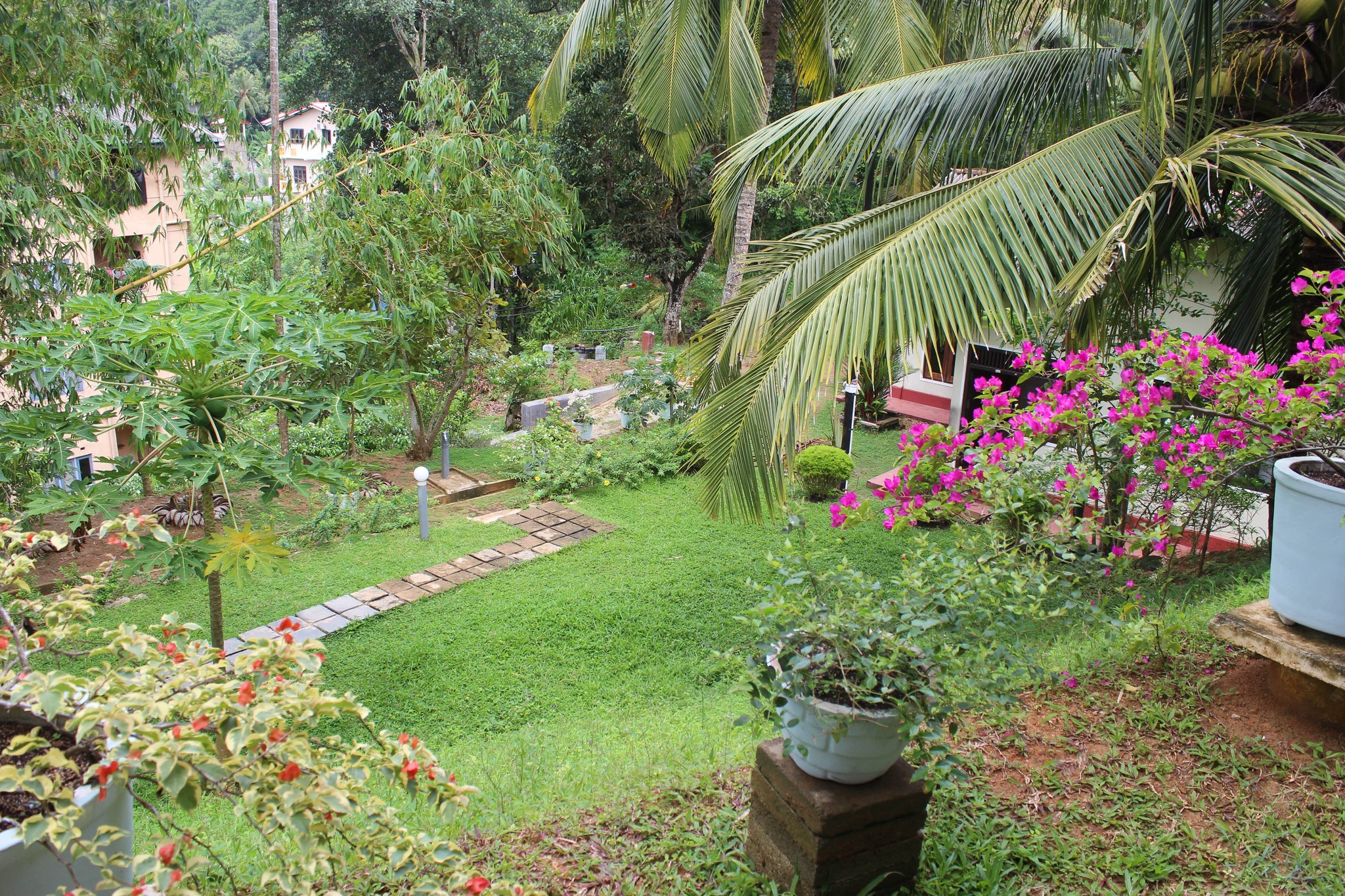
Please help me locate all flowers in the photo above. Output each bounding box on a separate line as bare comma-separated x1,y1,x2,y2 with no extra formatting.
568,388,598,431
603,399,641,411
826,267,1345,660
0,507,525,896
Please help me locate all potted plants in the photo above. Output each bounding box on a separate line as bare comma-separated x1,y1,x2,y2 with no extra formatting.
710,499,1063,788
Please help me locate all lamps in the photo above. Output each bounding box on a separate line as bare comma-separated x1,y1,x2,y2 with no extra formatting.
413,466,430,482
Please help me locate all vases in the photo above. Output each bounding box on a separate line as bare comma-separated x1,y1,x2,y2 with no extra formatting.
620,408,635,429
573,419,593,440
0,700,134,896
1268,457,1345,637
659,403,676,419
